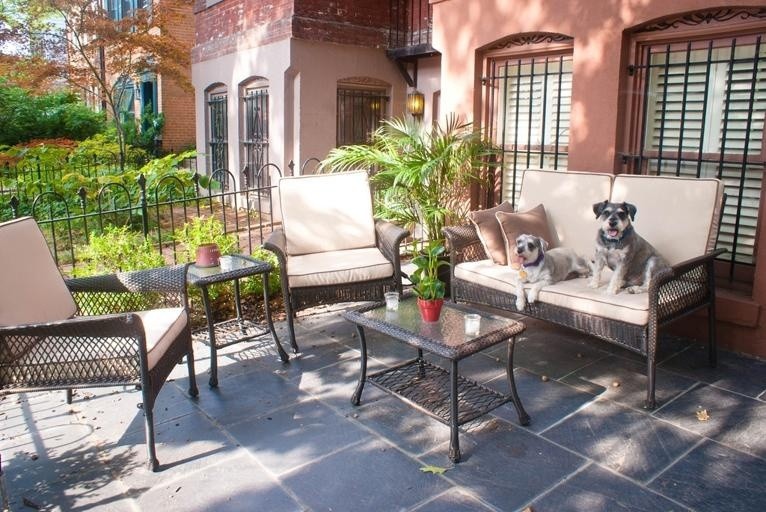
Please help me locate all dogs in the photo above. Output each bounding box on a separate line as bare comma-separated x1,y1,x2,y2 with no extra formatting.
512,232,593,313
588,200,672,297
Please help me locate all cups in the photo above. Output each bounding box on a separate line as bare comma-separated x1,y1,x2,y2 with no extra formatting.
384,292,398,310
464,313,482,336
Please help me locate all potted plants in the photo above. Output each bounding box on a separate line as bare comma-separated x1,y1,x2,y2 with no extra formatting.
317,116,503,298
409,238,451,322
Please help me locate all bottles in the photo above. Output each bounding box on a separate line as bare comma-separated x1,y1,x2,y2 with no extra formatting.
197,244,220,268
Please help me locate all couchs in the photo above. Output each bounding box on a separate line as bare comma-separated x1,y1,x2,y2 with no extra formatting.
442,167,729,409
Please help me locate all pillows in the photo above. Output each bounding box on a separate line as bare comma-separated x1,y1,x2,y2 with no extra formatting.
469,200,514,266
496,204,552,269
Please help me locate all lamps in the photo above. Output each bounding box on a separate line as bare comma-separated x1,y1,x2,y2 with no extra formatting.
408,92,424,123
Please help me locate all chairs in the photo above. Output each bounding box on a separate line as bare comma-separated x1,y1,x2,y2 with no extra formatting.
0,216,200,472
261,170,409,351
